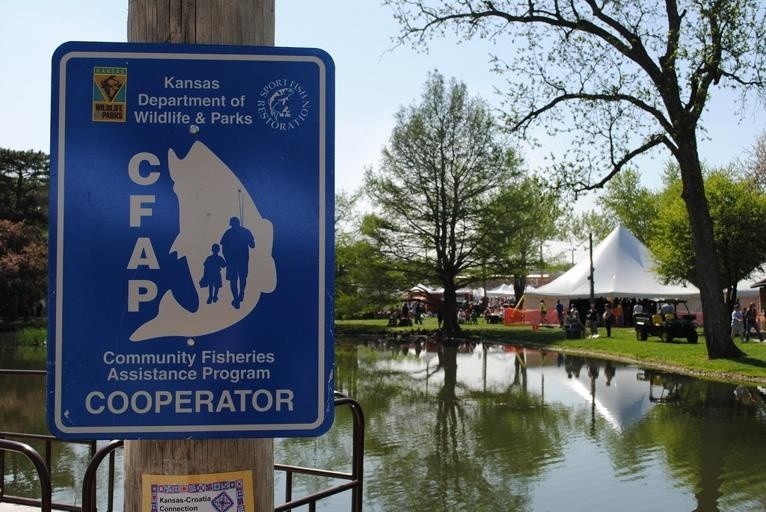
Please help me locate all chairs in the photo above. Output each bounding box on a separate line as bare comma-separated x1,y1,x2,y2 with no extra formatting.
665,313,673,320
652,314,663,326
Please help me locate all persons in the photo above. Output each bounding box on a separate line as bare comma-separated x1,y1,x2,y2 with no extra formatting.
564,303,586,339
555,299,564,327
388,338,422,359
730,304,747,344
602,304,612,337
745,302,766,344
557,351,617,387
741,307,747,329
395,296,518,325
662,301,674,315
539,299,549,323
632,301,644,316
587,303,599,339
617,301,626,327
